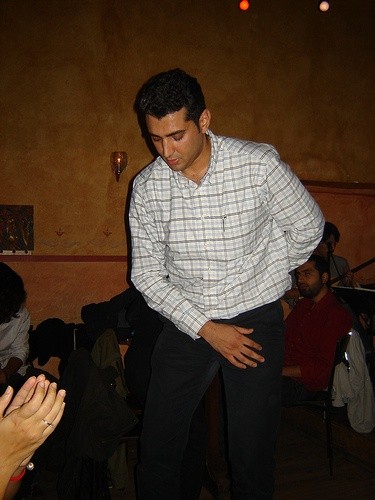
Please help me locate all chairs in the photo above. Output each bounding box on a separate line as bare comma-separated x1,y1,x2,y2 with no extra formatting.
281,327,358,476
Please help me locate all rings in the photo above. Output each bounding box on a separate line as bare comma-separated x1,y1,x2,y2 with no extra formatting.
42,418,56,428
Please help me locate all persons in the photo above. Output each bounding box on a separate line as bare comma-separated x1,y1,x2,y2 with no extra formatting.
129,70,326,500
0,221,356,500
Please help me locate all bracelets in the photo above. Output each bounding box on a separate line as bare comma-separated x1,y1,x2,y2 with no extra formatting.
10,462,35,481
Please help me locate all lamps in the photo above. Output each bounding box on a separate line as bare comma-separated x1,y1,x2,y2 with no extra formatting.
110,151,128,182
